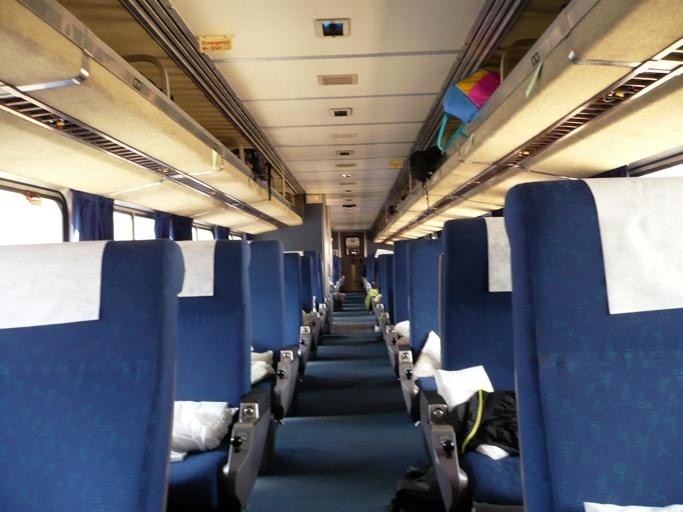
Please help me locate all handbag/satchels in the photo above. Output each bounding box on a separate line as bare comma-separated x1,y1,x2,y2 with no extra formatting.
409,146,440,181
443,68,499,122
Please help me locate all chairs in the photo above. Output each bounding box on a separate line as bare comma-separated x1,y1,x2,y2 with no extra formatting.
362,241,442,419
3,238,185,512
504,177,682,511
167,240,272,512
420,218,524,512
242,233,345,476
